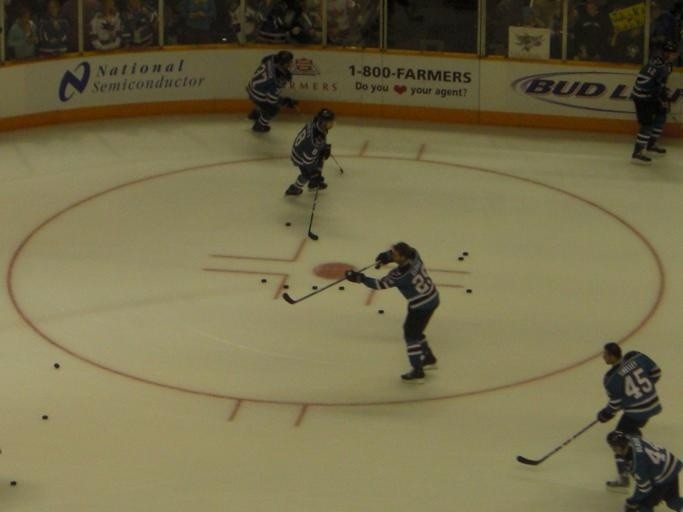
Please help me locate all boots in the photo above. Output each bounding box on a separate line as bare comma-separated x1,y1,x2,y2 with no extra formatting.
418,348,436,365
630,142,653,161
605,473,629,487
401,368,425,380
646,136,666,154
306,176,328,189
247,108,271,133
284,184,304,195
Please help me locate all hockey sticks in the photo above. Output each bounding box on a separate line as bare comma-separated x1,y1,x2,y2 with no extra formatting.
330,154,344,175
662,88,682,101
308,185,319,241
282,260,381,304
517,421,599,465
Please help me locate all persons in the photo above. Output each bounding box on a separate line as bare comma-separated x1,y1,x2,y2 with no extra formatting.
344,241,440,383
607,431,683,512
177,2,219,44
242,51,299,134
544,2,680,70
222,2,380,49
88,1,159,51
8,2,71,58
517,10,546,28
490,2,524,55
597,343,662,494
283,107,336,196
629,40,682,162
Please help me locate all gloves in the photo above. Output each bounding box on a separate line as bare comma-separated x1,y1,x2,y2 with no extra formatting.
597,405,614,424
284,96,298,110
345,270,365,285
322,142,332,160
372,252,392,268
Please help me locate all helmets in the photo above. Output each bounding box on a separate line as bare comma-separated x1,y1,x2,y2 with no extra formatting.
392,242,412,256
273,50,294,65
607,430,631,444
660,37,678,52
316,109,334,122
